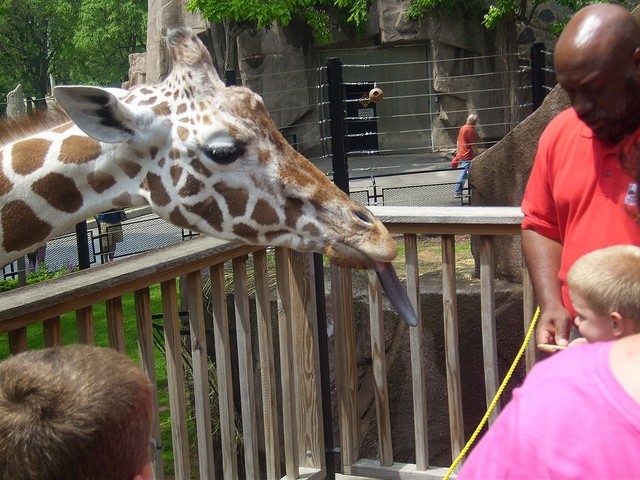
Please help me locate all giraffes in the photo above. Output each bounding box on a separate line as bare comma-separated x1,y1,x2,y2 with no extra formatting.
0,26,418,328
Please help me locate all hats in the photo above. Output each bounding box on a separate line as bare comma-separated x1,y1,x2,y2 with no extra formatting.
466,114,477,125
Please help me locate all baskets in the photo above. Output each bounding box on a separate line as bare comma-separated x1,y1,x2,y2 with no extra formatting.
147,305,259,442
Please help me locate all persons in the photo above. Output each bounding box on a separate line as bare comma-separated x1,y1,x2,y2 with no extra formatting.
27,244,47,276
451,332,639,480
1,344,158,480
453,113,480,199
518,2,639,356
93,206,128,263
566,244,639,349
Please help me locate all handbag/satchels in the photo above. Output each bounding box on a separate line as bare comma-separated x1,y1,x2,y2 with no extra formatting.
452,156,460,168
